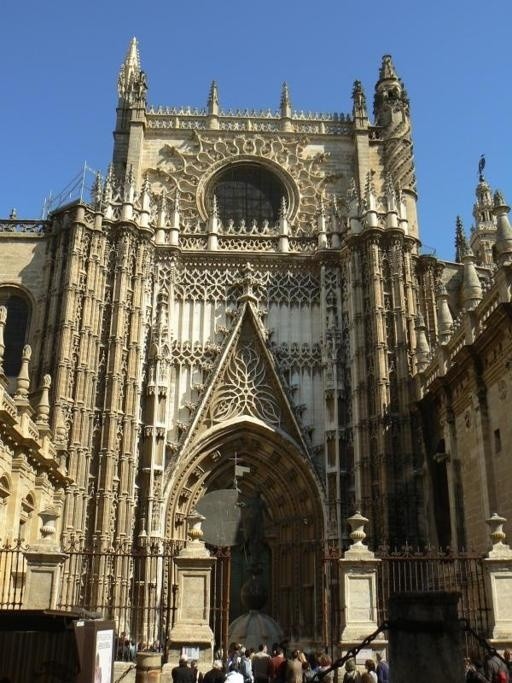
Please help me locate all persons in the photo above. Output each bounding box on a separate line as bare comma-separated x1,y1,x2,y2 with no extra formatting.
461,644,512,683
114,628,389,682
